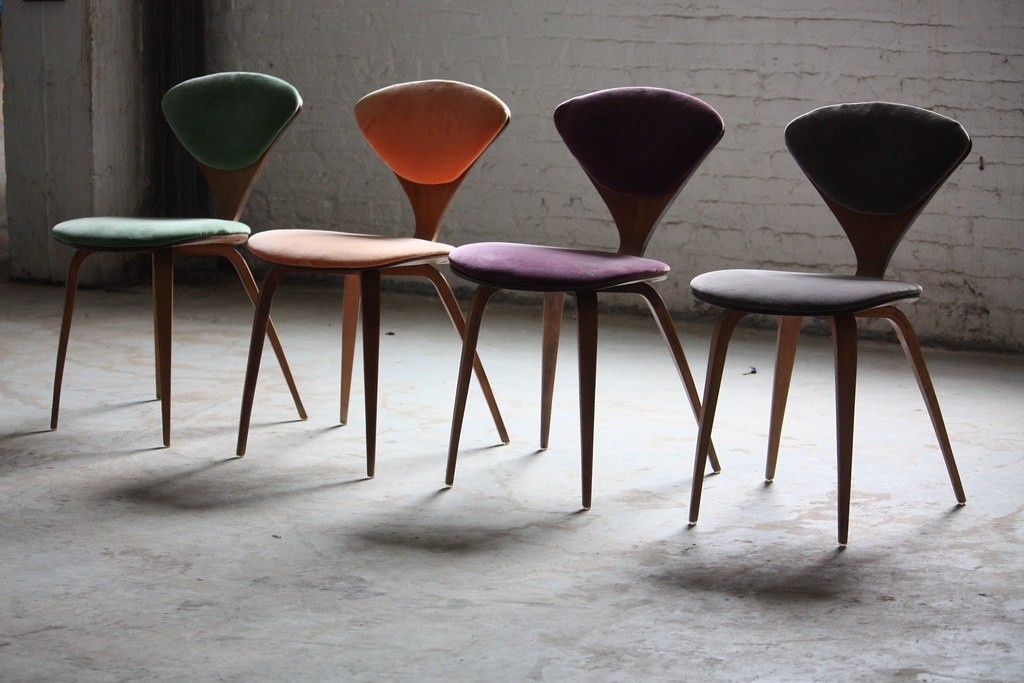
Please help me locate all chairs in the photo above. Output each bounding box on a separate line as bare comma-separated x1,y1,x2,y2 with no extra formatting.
687,101,972,549
236,79,511,478
51,72,308,447
444,86,726,510
